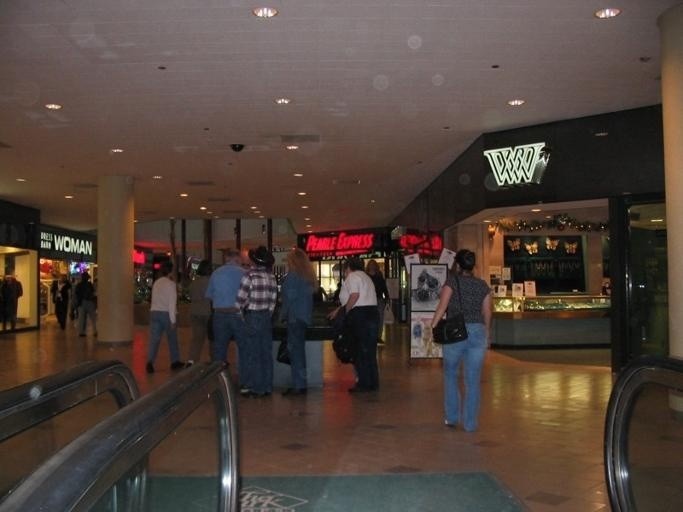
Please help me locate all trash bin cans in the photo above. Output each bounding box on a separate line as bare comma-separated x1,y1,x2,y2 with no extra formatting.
397,304,407,322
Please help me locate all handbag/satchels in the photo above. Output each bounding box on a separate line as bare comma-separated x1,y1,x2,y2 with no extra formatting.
432,312,469,345
382,301,395,325
277,335,292,364
332,305,353,365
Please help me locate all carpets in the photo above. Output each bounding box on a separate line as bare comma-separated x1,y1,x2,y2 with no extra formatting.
3,460,535,510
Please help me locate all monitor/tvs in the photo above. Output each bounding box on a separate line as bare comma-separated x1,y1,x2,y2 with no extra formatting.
68,262,94,279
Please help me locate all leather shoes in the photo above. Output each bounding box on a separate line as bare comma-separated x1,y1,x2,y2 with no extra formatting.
237,384,382,399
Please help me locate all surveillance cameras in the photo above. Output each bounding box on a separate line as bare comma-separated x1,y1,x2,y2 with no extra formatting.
231,144,244,152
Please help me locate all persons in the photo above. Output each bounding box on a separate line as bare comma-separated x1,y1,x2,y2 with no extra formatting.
326,257,379,393
318,280,328,302
146,262,192,372
74,272,97,337
366,260,389,344
431,249,491,433
0,276,23,330
187,260,213,368
277,248,320,396
50,274,72,338
234,246,278,398
204,247,250,395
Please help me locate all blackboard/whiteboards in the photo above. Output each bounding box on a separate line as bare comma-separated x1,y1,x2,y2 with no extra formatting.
386,278,399,300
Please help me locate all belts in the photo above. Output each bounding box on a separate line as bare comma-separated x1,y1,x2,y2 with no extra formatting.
214,307,241,314
244,308,269,313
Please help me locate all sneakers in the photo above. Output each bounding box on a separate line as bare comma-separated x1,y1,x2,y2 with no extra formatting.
146,363,155,374
168,360,195,371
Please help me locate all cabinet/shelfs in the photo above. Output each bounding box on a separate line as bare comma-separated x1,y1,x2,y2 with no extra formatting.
490,292,612,349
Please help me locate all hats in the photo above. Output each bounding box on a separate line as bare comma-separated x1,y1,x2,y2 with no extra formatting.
248,245,275,267
